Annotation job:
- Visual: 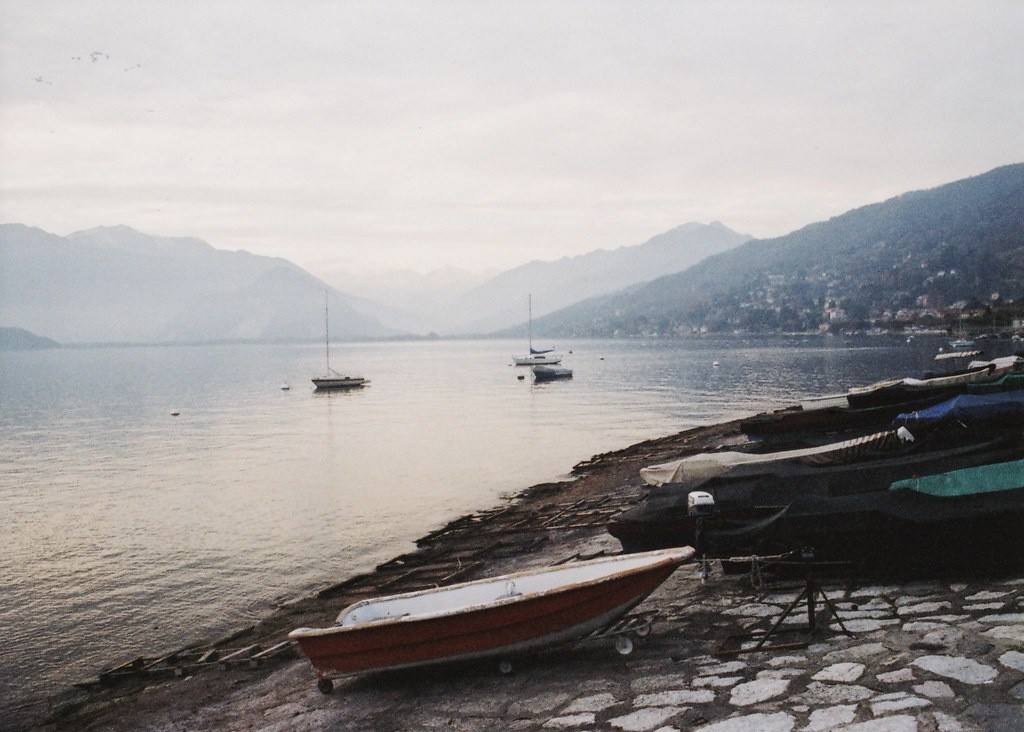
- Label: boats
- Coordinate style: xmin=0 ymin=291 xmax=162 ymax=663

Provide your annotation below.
xmin=605 ymin=309 xmax=1024 ymax=560
xmin=286 ymin=545 xmax=696 ymax=679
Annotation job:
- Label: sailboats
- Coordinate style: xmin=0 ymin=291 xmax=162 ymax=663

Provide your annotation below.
xmin=512 ymin=294 xmax=572 ymax=378
xmin=312 ymin=288 xmax=370 ymax=389
